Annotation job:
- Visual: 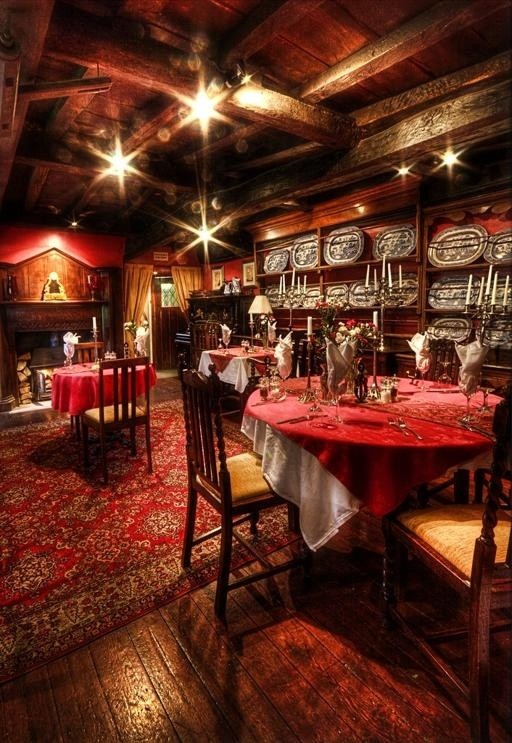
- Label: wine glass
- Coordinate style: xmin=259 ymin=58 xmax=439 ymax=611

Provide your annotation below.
xmin=457 ymin=371 xmax=496 ymax=424
xmin=63 ymin=344 xmax=74 ymax=369
xmin=437 ymin=362 xmax=452 ymax=385
xmin=307 ymin=377 xmax=349 ymax=425
xmin=217 ymin=338 xmax=224 ymax=352
xmin=417 ymin=355 xmax=432 ymax=390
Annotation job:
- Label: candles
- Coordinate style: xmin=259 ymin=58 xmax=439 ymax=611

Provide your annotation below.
xmin=279 ymin=268 xmax=307 ymax=294
xmin=365 ymin=255 xmax=402 ymax=290
xmin=92 ymin=317 xmax=97 ymax=331
xmin=466 ymin=265 xmax=510 ymax=305
xmin=250 ymin=314 xmax=253 ymax=322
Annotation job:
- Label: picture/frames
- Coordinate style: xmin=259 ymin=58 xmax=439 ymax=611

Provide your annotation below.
xmin=210 ymin=265 xmax=224 ymax=292
xmin=240 ymin=258 xmax=257 ymax=290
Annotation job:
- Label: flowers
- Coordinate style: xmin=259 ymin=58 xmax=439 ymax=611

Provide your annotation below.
xmin=254 ymin=315 xmax=276 ymax=340
xmin=309 ymin=299 xmax=383 ymax=362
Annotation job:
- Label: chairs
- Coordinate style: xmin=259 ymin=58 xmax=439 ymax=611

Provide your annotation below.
xmin=81 ymin=357 xmax=152 ymax=484
xmin=190 ymin=322 xmax=308 ymax=420
xmin=416 ymin=339 xmax=471 ymax=387
xmin=72 ymin=342 xmax=104 ymax=363
xmin=382 ymin=397 xmax=511 ymax=743
xmin=178 ymin=352 xmax=313 ymax=618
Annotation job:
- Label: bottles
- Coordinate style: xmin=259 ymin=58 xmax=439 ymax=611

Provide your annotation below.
xmin=259 ymin=358 xmax=286 ymax=403
xmin=241 ymin=340 xmax=249 ymax=352
xmin=353 ymin=364 xmax=369 ymax=401
xmin=105 ymin=349 xmax=116 ymax=359
xmin=381 ymin=376 xmax=400 ymax=405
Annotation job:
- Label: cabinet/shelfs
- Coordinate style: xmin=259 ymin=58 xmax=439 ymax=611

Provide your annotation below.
xmin=245 ymin=173 xmax=420 ymax=372
xmin=423 ymin=187 xmax=512 ymax=392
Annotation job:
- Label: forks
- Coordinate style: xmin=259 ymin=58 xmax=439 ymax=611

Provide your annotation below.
xmin=387 ymin=416 xmax=423 ymax=441
